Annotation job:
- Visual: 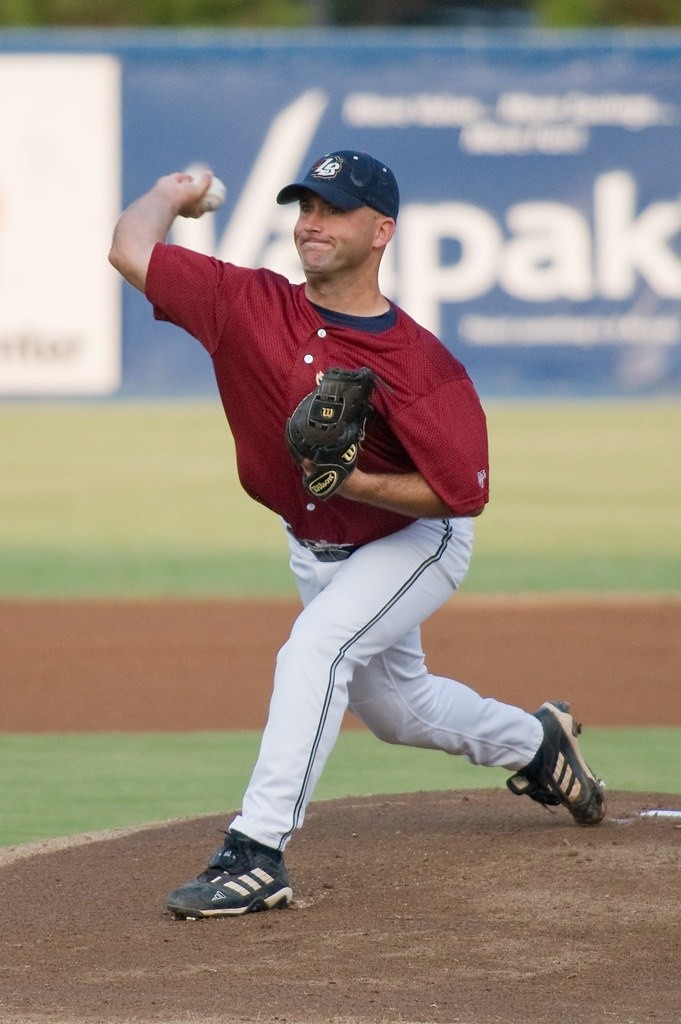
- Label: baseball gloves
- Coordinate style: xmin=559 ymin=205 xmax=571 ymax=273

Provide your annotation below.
xmin=285 ymin=366 xmax=376 ymax=500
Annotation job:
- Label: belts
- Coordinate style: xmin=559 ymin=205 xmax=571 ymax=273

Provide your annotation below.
xmin=286 ymin=526 xmax=363 ymax=562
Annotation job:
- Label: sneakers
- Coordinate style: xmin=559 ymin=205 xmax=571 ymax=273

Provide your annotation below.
xmin=506 ymin=700 xmax=607 ymax=826
xmin=167 ymin=829 xmax=293 ymax=918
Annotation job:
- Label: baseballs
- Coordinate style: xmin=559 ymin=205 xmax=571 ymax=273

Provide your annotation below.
xmin=193 ymin=176 xmax=226 ymax=211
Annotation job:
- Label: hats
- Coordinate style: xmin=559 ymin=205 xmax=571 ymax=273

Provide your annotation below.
xmin=277 ymin=149 xmax=400 ymax=220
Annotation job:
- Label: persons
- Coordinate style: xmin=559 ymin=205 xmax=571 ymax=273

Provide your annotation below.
xmin=110 ymin=149 xmax=607 ymax=918
